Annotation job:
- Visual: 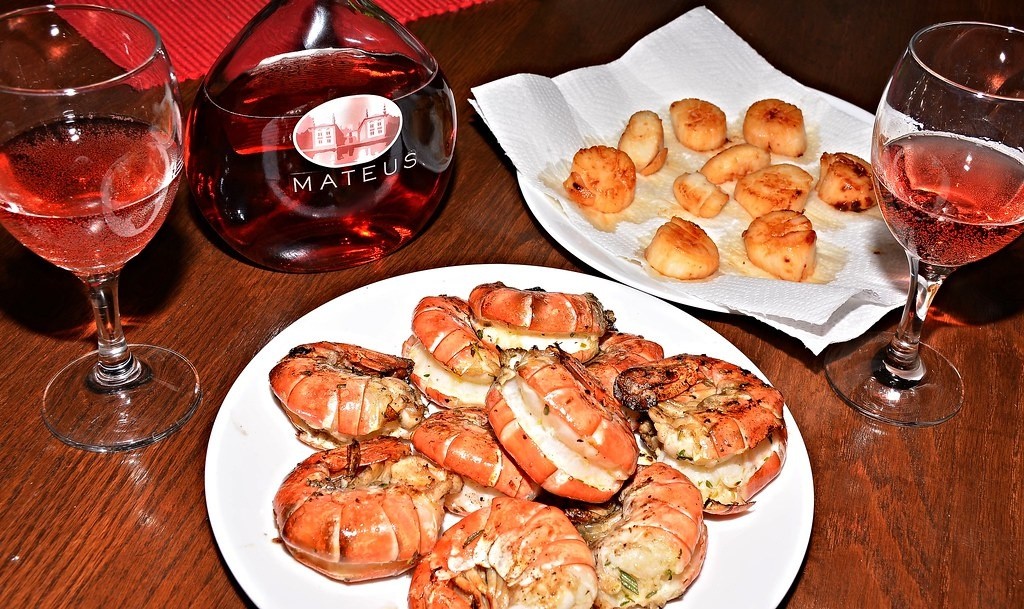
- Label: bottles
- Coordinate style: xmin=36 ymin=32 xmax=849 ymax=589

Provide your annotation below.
xmin=183 ymin=0 xmax=457 ymax=275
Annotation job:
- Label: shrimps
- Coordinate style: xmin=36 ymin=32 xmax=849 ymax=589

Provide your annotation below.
xmin=265 ymin=280 xmax=789 ymax=609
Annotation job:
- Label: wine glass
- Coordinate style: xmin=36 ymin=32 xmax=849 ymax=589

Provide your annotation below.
xmin=0 ymin=4 xmax=203 ymax=450
xmin=825 ymin=21 xmax=1024 ymax=428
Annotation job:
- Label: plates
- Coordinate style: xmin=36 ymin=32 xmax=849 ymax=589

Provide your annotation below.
xmin=204 ymin=263 xmax=815 ymax=609
xmin=517 ymin=87 xmax=877 ymax=316
